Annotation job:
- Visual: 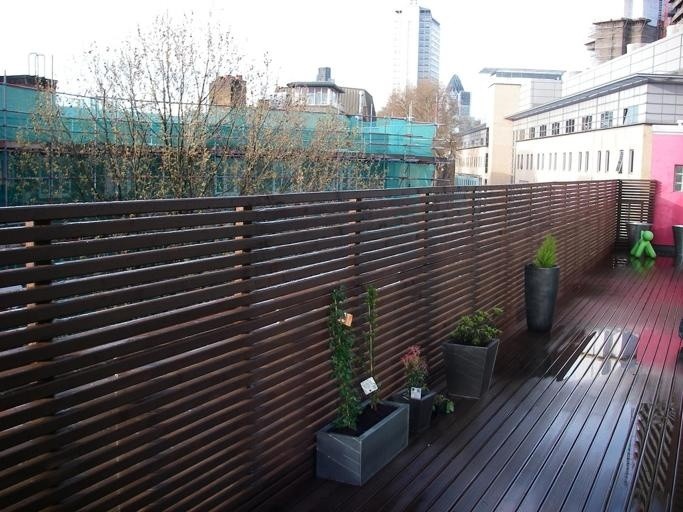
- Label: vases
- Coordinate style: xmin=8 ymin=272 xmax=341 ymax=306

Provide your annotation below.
xmin=672 ymin=225 xmax=683 ymax=256
xmin=627 ymin=223 xmax=653 ymax=256
xmin=400 ymin=390 xmax=436 ymax=434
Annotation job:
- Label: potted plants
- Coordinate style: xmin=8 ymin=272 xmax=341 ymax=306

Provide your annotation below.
xmin=525 ymin=235 xmax=561 ymax=332
xmin=442 ymin=305 xmax=504 ymax=400
xmin=317 ymin=280 xmax=409 ymax=487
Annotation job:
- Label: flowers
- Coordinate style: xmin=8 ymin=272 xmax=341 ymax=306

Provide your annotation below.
xmin=400 ymin=345 xmax=430 ymax=399
